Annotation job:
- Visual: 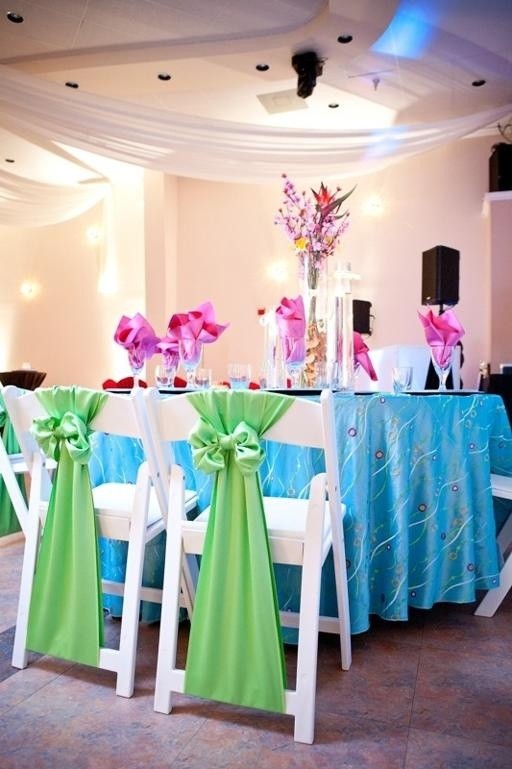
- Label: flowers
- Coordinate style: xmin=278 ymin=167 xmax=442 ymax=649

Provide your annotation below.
xmin=271 ymin=172 xmax=358 ymax=271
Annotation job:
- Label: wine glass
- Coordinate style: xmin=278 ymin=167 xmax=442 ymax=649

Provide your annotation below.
xmin=164 ymin=349 xmax=180 ymax=389
xmin=284 ymin=336 xmax=307 ymax=389
xmin=125 ymin=349 xmax=146 ymax=396
xmin=177 ymin=339 xmax=205 ymax=389
xmin=429 ymin=344 xmax=454 ymax=390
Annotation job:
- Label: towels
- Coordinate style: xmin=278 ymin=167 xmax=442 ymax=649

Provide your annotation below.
xmin=113 ymin=293 xmax=466 ymax=383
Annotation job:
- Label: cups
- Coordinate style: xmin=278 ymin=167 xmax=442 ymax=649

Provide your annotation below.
xmin=228 ymin=363 xmax=252 ymax=390
xmin=392 ymin=366 xmax=414 ymax=394
xmin=154 ymin=363 xmax=178 ymax=388
xmin=314 ymin=358 xmax=340 ymax=390
xmin=193 ymin=367 xmax=212 ymax=389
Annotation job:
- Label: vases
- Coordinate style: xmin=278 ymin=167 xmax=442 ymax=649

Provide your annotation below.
xmin=298 ymin=268 xmax=331 ymax=390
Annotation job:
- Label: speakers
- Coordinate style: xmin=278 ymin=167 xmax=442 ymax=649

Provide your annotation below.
xmin=422 ymin=245 xmax=459 ymax=305
xmin=352 ymin=299 xmax=372 ymax=335
xmin=489 ymin=145 xmax=512 ymax=191
